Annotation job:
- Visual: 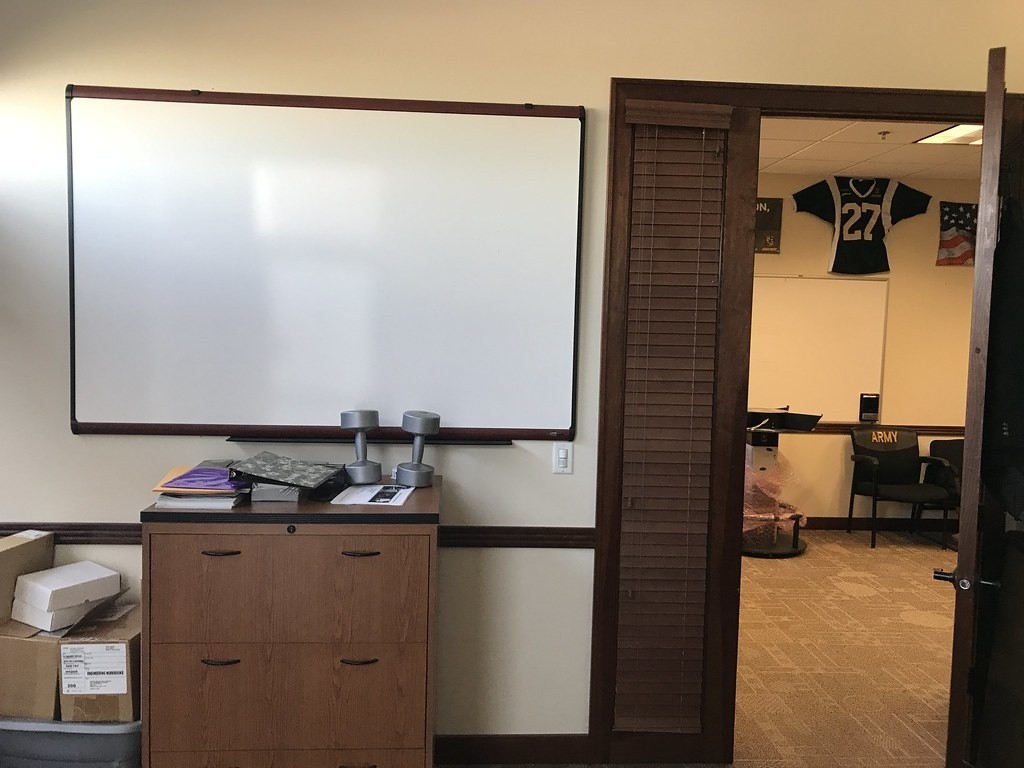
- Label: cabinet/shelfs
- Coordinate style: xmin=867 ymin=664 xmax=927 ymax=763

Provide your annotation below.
xmin=139 ymin=474 xmax=443 ymax=768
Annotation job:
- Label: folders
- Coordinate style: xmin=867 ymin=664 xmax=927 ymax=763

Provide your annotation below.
xmin=228 ymin=451 xmax=338 ymax=489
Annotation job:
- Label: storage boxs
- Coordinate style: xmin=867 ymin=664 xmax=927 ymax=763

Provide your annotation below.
xmin=746 ymin=428 xmax=779 ymax=514
xmin=59 ymin=603 xmax=142 ymax=722
xmin=0 ymin=720 xmax=142 ymax=768
xmin=0 ymin=617 xmax=73 ymax=721
xmin=0 ymin=530 xmax=57 ymax=627
xmin=10 ymin=596 xmax=113 ymax=631
xmin=14 ymin=560 xmax=120 ymax=611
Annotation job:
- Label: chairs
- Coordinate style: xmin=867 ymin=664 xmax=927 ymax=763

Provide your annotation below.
xmin=846 ymin=425 xmax=951 ymax=552
xmin=914 ymin=439 xmax=964 ymax=533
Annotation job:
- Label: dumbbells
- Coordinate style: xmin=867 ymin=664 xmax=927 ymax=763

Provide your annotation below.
xmin=396 ymin=410 xmax=440 ymax=487
xmin=341 ymin=411 xmax=383 ymax=486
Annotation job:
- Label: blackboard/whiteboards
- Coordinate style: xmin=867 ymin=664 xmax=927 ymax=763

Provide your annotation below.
xmin=65 ymin=82 xmax=585 ymax=444
xmin=747 ymin=272 xmax=891 ymax=426
